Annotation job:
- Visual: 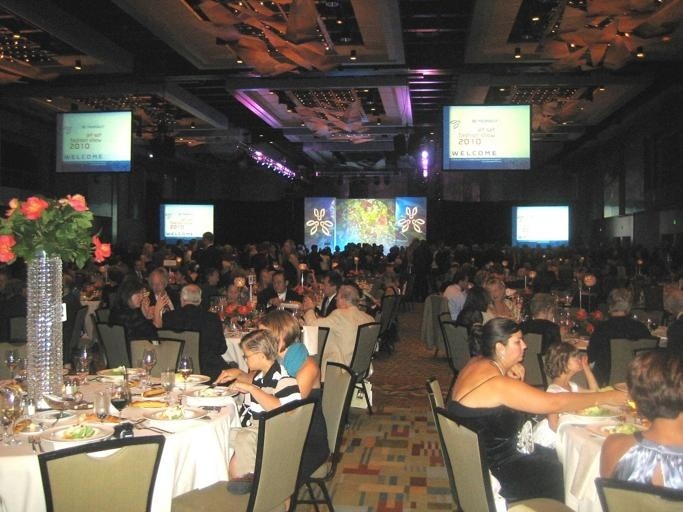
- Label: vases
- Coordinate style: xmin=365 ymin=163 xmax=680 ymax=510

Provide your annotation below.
xmin=26 ymin=260 xmax=63 ymax=403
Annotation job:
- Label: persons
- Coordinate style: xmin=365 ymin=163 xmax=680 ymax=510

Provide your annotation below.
xmin=585 ymin=286 xmax=660 ymax=386
xmin=0 ymin=257 xmax=29 ymax=343
xmin=443 ymin=273 xmax=469 ymax=322
xmin=662 ymin=289 xmax=682 ymax=356
xmin=455 ymin=287 xmax=488 ymax=358
xmin=514 ymin=293 xmax=562 ymax=359
xmin=109 ymin=275 xmax=169 ymax=343
xmin=219 ymin=239 xmax=407 ymax=322
xmin=141 ymin=254 xmax=154 ymax=272
xmin=62 ymin=242 xmax=110 ymax=375
xmin=128 ymin=257 xmax=148 ymax=281
xmin=483 ymin=277 xmax=521 ymax=324
xmin=302 ymin=286 xmax=374 ymax=384
xmin=199 ymin=269 xmax=225 ymax=312
xmin=200 ymin=232 xmax=220 ymax=269
xmin=529 ymin=341 xmax=598 ymax=449
xmin=149 ymin=267 xmax=180 ymax=315
xmin=211 ymin=311 xmax=329 ymax=512
xmin=110 ymin=238 xmax=201 ymax=262
xmin=407 ymin=237 xmax=432 ymax=302
xmin=445 ymin=316 xmax=629 ymax=505
xmin=160 ymin=284 xmax=239 ymax=385
xmin=426 ymin=235 xmax=683 ymax=291
xmin=599 ymin=349 xmax=682 ymax=492
xmin=229 ymin=329 xmax=303 ymax=482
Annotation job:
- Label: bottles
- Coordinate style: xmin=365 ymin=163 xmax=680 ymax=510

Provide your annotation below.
xmin=72 ymin=381 xmax=78 ymax=397
xmin=27 ymin=399 xmax=36 ymax=418
xmin=20 ymin=400 xmax=27 ymax=417
xmin=65 ymin=381 xmax=72 ymax=395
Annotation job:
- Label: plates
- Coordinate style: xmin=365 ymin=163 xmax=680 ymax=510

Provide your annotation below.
xmin=143 ymin=405 xmax=212 ymax=424
xmin=185 ymin=385 xmax=239 ymax=400
xmin=97 ymin=367 xmax=146 ymax=383
xmin=39 ymin=423 xmax=117 ymax=443
xmin=601 ymin=424 xmax=649 ymax=435
xmin=176 ymin=372 xmax=212 ymax=385
xmin=32 ymin=409 xmax=75 ymax=423
xmin=614 ymin=381 xmax=628 ymax=391
xmin=563 ymin=404 xmax=624 ymax=420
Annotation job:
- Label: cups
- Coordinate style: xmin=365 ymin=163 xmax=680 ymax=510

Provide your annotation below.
xmin=110 ymin=385 xmax=127 ymax=423
xmin=95 ymin=391 xmax=107 ymax=424
xmin=70 ymin=352 xmax=94 ymax=385
xmin=2 ymin=388 xmax=23 ymax=446
xmin=237 ymin=314 xmax=246 ymax=330
xmin=178 ymin=356 xmax=193 ymax=389
xmin=159 ymin=368 xmax=175 ymax=404
xmin=293 ymin=308 xmax=302 ymax=323
xmin=141 ymin=349 xmax=158 ymax=382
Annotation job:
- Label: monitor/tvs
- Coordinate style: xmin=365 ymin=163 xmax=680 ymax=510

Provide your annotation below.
xmin=441 ymin=104 xmax=532 ymax=171
xmin=56 ymin=110 xmax=133 ymax=175
xmin=159 ymin=202 xmax=215 ymax=241
xmin=510 ymin=203 xmax=573 ymax=249
xmin=303 ymin=196 xmax=428 ymax=256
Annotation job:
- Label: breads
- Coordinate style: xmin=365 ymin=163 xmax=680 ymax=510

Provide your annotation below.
xmin=128 ymin=379 xmax=139 ymax=387
xmin=85 ymin=413 xmax=120 ymax=423
xmin=128 ymin=400 xmax=169 ymax=409
xmin=143 ymin=386 xmax=166 ymax=399
xmin=13 ymin=418 xmax=32 ymax=433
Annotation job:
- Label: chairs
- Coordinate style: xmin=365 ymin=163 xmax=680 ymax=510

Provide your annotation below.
xmin=8 ymin=315 xmax=26 ymax=341
xmin=291 ymin=360 xmax=357 ymax=512
xmin=94 ymin=322 xmax=130 ymax=370
xmin=299 ymin=326 xmax=332 ymax=397
xmin=425 ymin=378 xmax=534 ymax=504
xmin=348 ymin=322 xmax=382 ymax=415
xmin=630 ymin=306 xmax=665 ymax=329
xmin=522 ymin=332 xmax=549 ymax=390
xmin=398 ymin=275 xmax=414 ymax=313
xmin=129 ymin=338 xmax=186 ymax=374
xmin=154 ymin=328 xmax=202 ymax=373
xmin=64 ymin=304 xmax=88 ymax=371
xmin=608 ymin=336 xmax=661 ymax=390
xmin=170 ymin=396 xmax=317 ymax=512
xmin=0 ymin=342 xmax=26 ymax=379
xmin=441 ymin=321 xmax=473 ymax=373
xmin=438 ymin=313 xmax=454 ymax=359
xmin=376 ymin=294 xmax=398 ymax=354
xmin=432 ymin=406 xmax=575 ymax=512
xmin=594 ymin=477 xmax=683 ymax=511
xmin=37 ymin=434 xmax=166 ymax=512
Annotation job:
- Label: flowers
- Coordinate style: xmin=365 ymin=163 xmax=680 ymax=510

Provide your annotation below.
xmin=569 ymin=308 xmax=605 ymax=336
xmin=222 ymin=302 xmax=254 ymax=329
xmin=0 ymin=196 xmax=111 ymax=259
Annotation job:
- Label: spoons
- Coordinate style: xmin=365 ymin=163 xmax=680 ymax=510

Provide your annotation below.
xmin=133 ymin=424 xmax=164 ymax=437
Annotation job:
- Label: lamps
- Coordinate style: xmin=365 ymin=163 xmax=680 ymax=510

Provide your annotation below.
xmin=600 ymin=85 xmax=605 ymax=91
xmin=14 ymin=28 xmax=20 ymax=40
xmin=636 ymin=47 xmax=643 ymax=58
xmin=190 ymin=121 xmax=196 ymax=129
xmin=351 ymin=49 xmax=357 ymax=61
xmin=515 ymin=47 xmax=522 ymax=58
xmin=337 ymin=16 xmax=342 ymax=25
xmin=74 ymin=59 xmax=83 ymax=72
xmin=533 ymin=12 xmax=540 ymax=22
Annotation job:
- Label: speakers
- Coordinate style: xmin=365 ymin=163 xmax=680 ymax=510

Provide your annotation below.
xmin=170 ymin=143 xmax=207 ymax=172
xmin=571 ymin=145 xmax=605 ymax=180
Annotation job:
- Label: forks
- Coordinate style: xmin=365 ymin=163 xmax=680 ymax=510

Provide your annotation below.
xmin=139 ymin=422 xmax=175 ymax=436
xmin=33 ymin=435 xmax=46 ymax=454
xmin=26 ymin=434 xmax=39 ymax=455
